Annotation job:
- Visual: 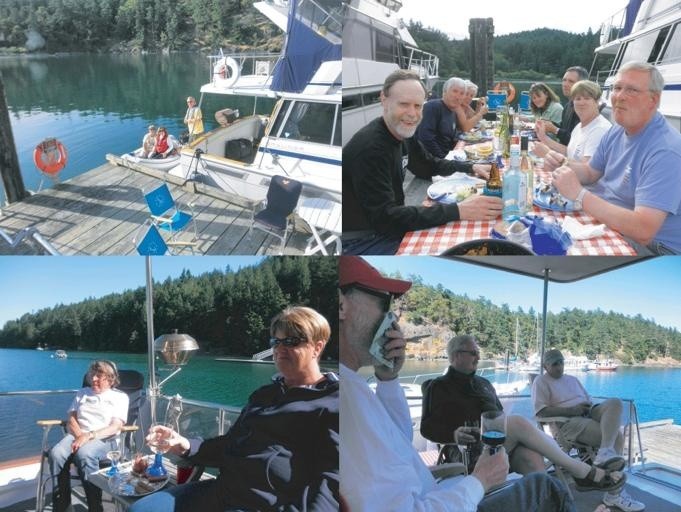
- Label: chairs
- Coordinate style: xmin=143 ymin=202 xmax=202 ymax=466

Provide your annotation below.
xmin=418 ymin=380 xmax=472 ymax=474
xmin=36 ymin=370 xmax=144 ymax=511
xmin=144 ymin=182 xmax=198 ymax=244
xmin=518 ymin=90 xmax=533 ymax=115
xmin=532 ymin=415 xmax=626 ymax=501
xmin=250 ymin=175 xmax=303 ymax=255
xmin=429 ymin=463 xmax=468 ymax=484
xmin=132 ymin=218 xmax=174 ymax=256
xmin=486 ymin=89 xmax=508 ymax=110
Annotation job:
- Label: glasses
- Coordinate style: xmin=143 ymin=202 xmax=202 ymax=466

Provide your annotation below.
xmin=342 ymin=285 xmax=396 ymax=314
xmin=452 ymin=349 xmax=481 ymax=357
xmin=269 ymin=335 xmax=308 ymax=349
xmin=612 ymin=85 xmax=656 ymax=98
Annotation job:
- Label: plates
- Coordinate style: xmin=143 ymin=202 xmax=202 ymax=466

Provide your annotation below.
xmin=107 ymin=470 xmax=170 ymax=498
xmin=454 ymin=150 xmax=498 ymax=164
xmin=427 ymin=176 xmax=486 ymax=206
xmin=458 ymin=131 xmax=493 ymax=142
xmin=528 ymin=150 xmax=543 ymax=160
xmin=533 ymin=185 xmax=581 ymax=212
xmin=439 ymin=238 xmax=537 ymax=256
xmin=521 ymin=131 xmax=540 ymax=141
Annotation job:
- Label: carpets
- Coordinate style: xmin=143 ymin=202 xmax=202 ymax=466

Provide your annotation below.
xmin=0 ymin=484 xmax=119 ymax=512
xmin=563 ymin=482 xmax=681 ymax=512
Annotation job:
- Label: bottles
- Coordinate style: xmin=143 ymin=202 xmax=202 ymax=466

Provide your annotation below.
xmin=508 ymin=107 xmax=515 ymax=116
xmin=519 ymin=134 xmax=533 ymax=211
xmin=486 ymin=161 xmax=502 ymax=220
xmin=501 ymin=134 xmax=527 ymax=223
xmin=499 ymin=112 xmax=510 ymax=159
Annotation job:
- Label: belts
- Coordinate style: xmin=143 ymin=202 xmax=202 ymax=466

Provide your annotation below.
xmin=343 ymin=228 xmax=381 ymax=240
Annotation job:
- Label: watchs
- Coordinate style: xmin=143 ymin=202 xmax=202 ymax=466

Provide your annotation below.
xmin=572 ymin=187 xmax=587 ymax=213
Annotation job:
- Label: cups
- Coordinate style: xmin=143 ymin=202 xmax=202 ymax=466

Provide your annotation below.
xmin=583 ymin=393 xmax=593 ymax=418
xmin=130 ymin=452 xmax=148 ymax=477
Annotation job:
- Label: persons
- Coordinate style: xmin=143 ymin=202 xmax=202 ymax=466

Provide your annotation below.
xmin=148 ymin=128 xmax=171 ymax=160
xmin=48 ymin=360 xmax=130 ymax=511
xmin=339 ymin=256 xmax=579 ymax=511
xmin=141 ymin=125 xmax=156 ymax=158
xmin=130 ymin=307 xmax=338 ymax=512
xmin=421 ymin=336 xmax=627 ymax=492
xmin=185 ymin=97 xmax=203 ymax=142
xmin=544 ymin=61 xmax=680 ymax=257
xmin=531 ymin=350 xmax=645 ymax=511
xmin=416 ymin=66 xmax=614 ymax=164
xmin=341 ymin=69 xmax=503 ymax=256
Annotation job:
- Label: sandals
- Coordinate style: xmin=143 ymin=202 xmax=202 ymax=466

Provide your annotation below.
xmin=574 ymin=464 xmax=628 ymax=492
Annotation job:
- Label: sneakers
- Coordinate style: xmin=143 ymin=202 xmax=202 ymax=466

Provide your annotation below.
xmin=601 ymin=488 xmax=646 ymax=511
xmin=593 ymin=447 xmax=627 ymax=474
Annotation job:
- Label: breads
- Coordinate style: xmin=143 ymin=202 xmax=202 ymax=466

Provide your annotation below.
xmin=478 ymin=144 xmax=493 ymax=156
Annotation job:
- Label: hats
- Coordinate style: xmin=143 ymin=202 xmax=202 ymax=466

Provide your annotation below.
xmin=340 ymin=255 xmax=412 ymax=297
xmin=542 ymin=348 xmax=564 ymax=367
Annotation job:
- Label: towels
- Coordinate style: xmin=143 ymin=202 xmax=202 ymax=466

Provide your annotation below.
xmin=368 ymin=312 xmax=399 ymax=372
xmin=530 ymin=215 xmax=573 ymax=256
xmin=562 ymin=213 xmax=608 ymax=245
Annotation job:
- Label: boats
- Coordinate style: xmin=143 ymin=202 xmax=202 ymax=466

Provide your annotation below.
xmin=127 ymin=140 xmax=181 ymax=169
xmin=54 ymin=347 xmax=69 ymax=361
xmin=182 ymin=0 xmax=341 ymax=234
xmin=35 ymin=341 xmax=49 ymax=351
xmin=361 ymin=365 xmax=532 ymax=448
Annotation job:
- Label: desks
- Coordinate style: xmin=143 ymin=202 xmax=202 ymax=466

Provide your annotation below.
xmin=298 ymin=198 xmax=342 ymax=257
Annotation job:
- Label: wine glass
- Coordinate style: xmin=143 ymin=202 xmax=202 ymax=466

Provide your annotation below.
xmin=106 ymin=438 xmax=122 ymax=475
xmin=464 ymin=418 xmax=479 ymax=452
xmin=143 ymin=421 xmax=172 ymax=482
xmin=496 ymin=106 xmax=505 ymax=119
xmin=479 ymin=411 xmax=507 ymax=455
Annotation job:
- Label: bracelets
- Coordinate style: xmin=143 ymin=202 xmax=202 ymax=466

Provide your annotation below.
xmin=89 ymin=431 xmax=94 ymax=438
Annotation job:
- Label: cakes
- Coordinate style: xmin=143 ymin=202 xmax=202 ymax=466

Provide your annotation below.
xmin=456 ymin=185 xmax=477 ymax=202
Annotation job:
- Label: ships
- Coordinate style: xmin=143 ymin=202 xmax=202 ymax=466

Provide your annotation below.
xmin=341 ymin=0 xmax=441 ymax=150
xmin=589 ymin=1 xmax=681 ymax=119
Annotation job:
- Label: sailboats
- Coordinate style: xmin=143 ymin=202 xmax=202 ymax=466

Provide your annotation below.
xmin=495 ymin=310 xmax=620 ymax=373
